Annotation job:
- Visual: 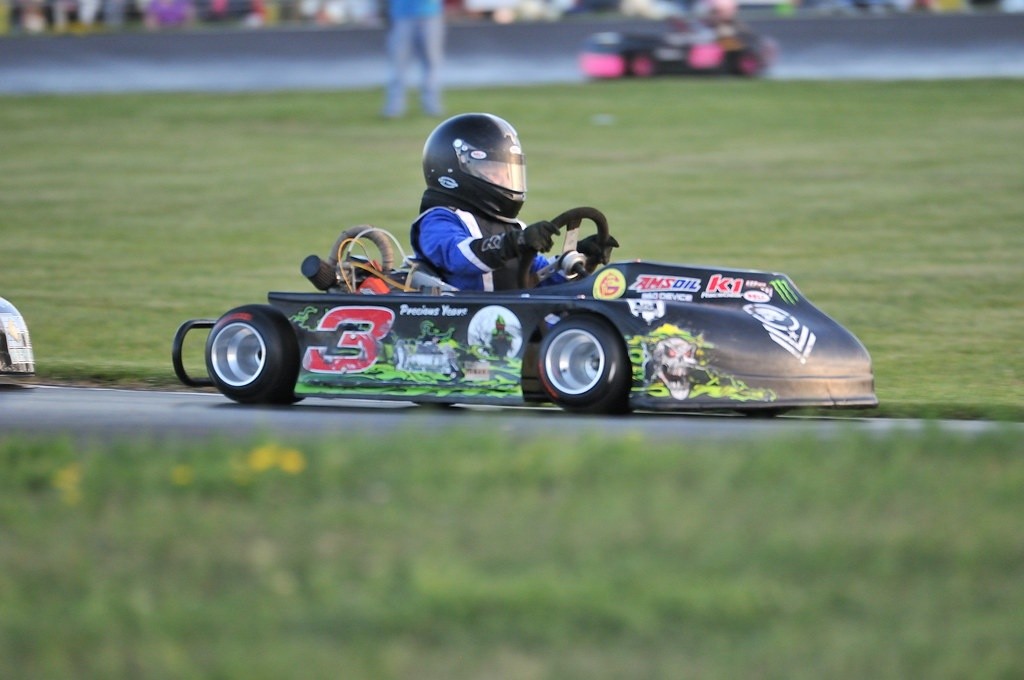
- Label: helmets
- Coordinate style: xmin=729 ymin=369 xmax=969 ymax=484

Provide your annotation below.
xmin=423 ymin=113 xmax=527 ymax=220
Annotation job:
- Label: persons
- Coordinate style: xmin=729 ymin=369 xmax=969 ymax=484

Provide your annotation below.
xmin=381 ymin=0 xmax=447 ymax=119
xmin=410 ymin=113 xmax=620 ymax=290
xmin=667 ymin=0 xmax=738 ymax=42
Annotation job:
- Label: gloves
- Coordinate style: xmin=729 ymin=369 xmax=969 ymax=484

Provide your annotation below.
xmin=576 ymin=233 xmax=619 ymax=266
xmin=507 ymin=220 xmax=560 ymax=258
